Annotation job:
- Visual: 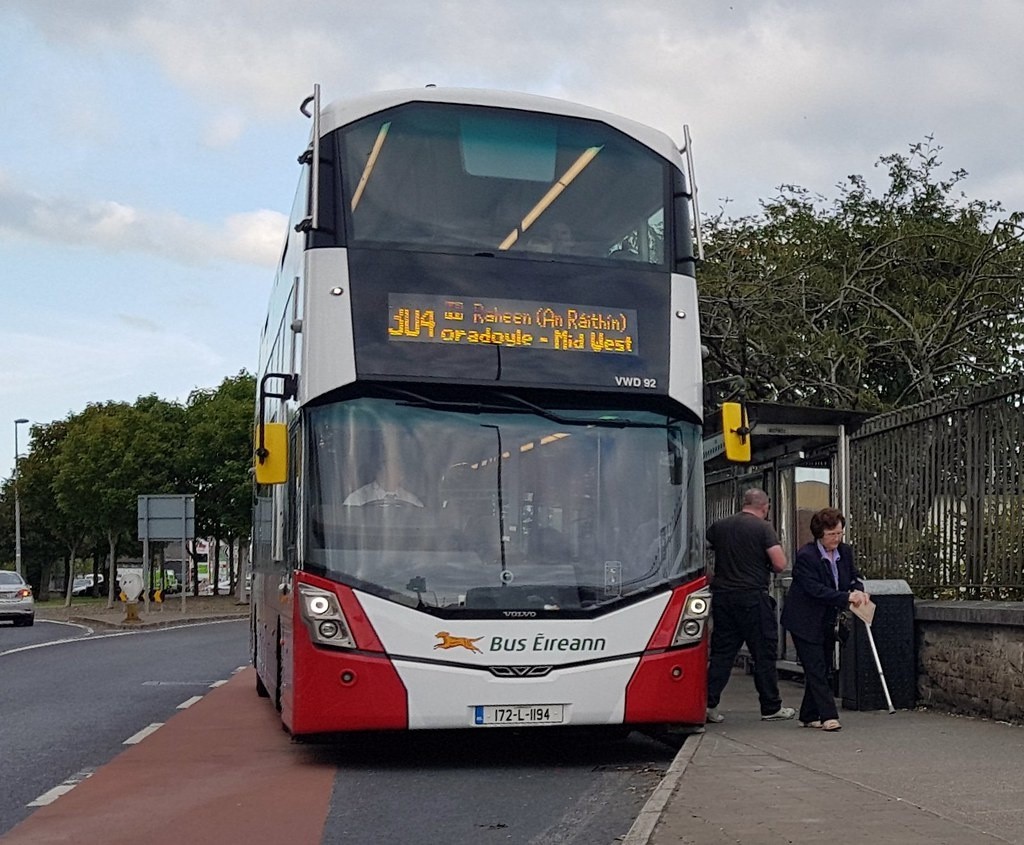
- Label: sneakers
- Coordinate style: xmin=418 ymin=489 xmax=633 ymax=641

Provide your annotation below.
xmin=706 ymin=704 xmax=725 ymax=722
xmin=761 ymin=708 xmax=795 ymax=720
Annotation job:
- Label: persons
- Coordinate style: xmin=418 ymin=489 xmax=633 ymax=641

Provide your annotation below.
xmin=780 ymin=508 xmax=871 ymax=732
xmin=704 ymin=488 xmax=789 ymax=723
xmin=343 ymin=451 xmax=427 ymax=509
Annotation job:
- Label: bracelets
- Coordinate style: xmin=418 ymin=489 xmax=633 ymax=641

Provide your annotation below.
xmin=823 ymin=544 xmax=834 ymax=552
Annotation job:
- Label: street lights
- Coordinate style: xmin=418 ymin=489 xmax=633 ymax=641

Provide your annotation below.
xmin=13 ymin=418 xmax=29 ymax=577
xmin=478 ymin=423 xmax=508 ymax=587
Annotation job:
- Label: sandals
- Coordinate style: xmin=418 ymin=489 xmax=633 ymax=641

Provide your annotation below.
xmin=798 ymin=720 xmax=823 ymax=727
xmin=823 ymin=719 xmax=842 ymax=730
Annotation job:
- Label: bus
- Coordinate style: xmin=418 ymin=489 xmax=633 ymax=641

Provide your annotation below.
xmin=244 ymin=80 xmax=764 ymax=738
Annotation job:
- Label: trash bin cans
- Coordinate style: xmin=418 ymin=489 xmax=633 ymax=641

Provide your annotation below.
xmin=840 ymin=579 xmax=916 ymax=710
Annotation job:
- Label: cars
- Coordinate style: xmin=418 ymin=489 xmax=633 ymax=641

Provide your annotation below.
xmin=0 ymin=569 xmax=36 ymax=627
xmin=70 ymin=566 xmax=145 ymax=598
